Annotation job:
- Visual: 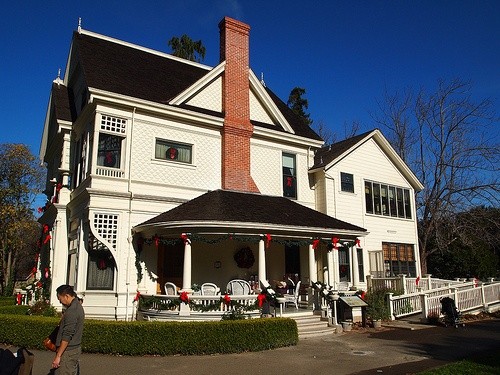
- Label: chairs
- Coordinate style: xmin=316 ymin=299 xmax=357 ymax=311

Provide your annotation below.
xmin=283 ymin=281 xmax=301 ymax=312
xmin=226 ymin=279 xmax=251 ymax=295
xmin=164 ymin=282 xmax=176 ymax=296
xmin=201 ymin=283 xmax=217 ymax=296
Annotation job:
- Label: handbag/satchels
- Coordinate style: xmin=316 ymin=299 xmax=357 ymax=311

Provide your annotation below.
xmin=43 ymin=325 xmax=60 ymax=352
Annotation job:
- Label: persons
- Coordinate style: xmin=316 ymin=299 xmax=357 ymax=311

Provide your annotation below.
xmin=16 ymin=292 xmax=22 ymax=305
xmin=51 ymin=284 xmax=85 ymax=375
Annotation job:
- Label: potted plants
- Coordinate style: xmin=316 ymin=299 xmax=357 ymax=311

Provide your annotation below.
xmin=192 ymin=283 xmax=202 ymax=296
xmin=368 ymin=290 xmax=385 ymax=328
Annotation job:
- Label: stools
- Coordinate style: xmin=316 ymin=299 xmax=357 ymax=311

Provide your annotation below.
xmin=274 ymin=298 xmax=286 ymax=318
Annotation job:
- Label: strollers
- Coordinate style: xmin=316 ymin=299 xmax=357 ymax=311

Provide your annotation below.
xmin=438 ymin=297 xmax=465 ymax=328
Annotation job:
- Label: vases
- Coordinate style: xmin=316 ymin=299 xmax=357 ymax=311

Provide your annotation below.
xmin=341 ymin=322 xmax=353 ymax=331
